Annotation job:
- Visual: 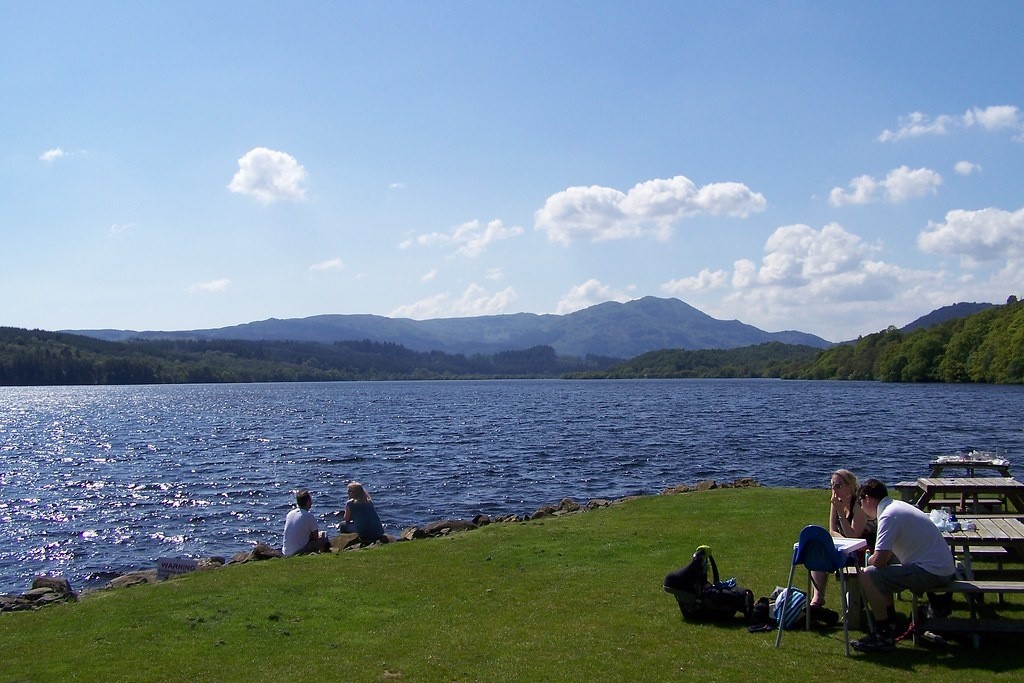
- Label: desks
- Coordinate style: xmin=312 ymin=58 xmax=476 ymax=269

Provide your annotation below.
xmin=941 ymin=516 xmax=1024 ymax=647
xmin=915 ymin=477 xmax=1024 ymax=514
xmin=928 ymin=458 xmax=1012 ymax=478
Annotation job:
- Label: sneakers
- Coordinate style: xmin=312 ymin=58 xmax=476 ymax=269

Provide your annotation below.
xmin=849 ymin=627 xmax=898 ymax=654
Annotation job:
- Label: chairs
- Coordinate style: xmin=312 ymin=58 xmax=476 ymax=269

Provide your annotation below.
xmin=775 ymin=525 xmax=874 ymax=657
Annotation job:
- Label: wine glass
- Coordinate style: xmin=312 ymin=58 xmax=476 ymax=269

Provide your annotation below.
xmin=955 ymin=451 xmax=961 ymax=462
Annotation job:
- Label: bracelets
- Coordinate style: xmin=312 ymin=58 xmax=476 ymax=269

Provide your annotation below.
xmin=839 ymin=514 xmax=845 ymax=519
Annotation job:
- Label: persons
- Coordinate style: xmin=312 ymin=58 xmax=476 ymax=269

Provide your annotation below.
xmin=850 ymin=479 xmax=956 ymax=650
xmin=803 ymin=469 xmax=883 ymax=612
xmin=340 ymin=481 xmax=385 ymax=542
xmin=282 ymin=491 xmax=328 ymax=557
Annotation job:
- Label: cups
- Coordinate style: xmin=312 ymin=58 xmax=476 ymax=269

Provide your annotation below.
xmin=942 ymin=506 xmax=951 ymax=517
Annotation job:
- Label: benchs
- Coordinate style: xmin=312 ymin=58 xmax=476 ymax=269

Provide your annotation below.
xmin=835 ymin=546 xmax=1024 ymax=646
xmin=927 ymin=499 xmax=1002 ymax=514
xmin=894 ymin=481 xmax=917 ymax=502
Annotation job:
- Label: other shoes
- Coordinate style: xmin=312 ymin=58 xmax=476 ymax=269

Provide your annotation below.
xmin=802 ymin=602 xmax=829 ymax=613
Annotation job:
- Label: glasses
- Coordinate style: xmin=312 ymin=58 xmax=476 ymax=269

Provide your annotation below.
xmin=857 ymin=496 xmax=866 ymax=505
xmin=829 ymin=481 xmax=845 ymax=489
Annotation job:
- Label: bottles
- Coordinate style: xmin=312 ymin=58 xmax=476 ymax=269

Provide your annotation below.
xmin=865 ymin=549 xmax=871 ymax=567
xmin=951 ymin=522 xmax=976 ymax=531
